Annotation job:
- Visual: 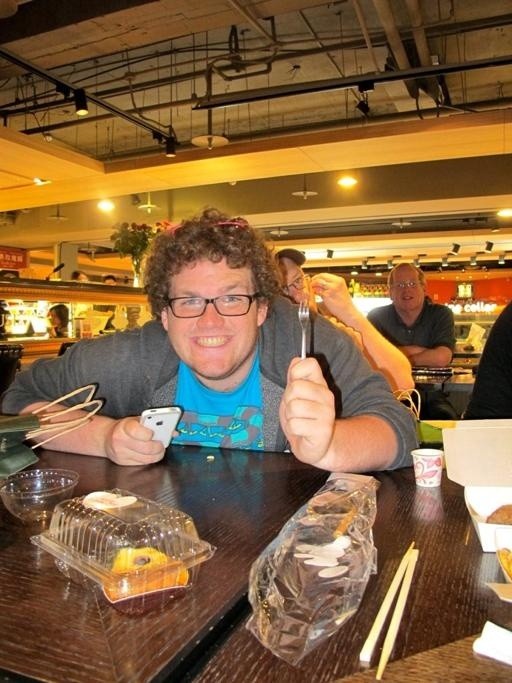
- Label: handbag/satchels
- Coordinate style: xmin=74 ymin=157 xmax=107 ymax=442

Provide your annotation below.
xmin=392 ymin=387 xmax=456 ymax=442
xmin=1 ymin=385 xmax=104 ymax=482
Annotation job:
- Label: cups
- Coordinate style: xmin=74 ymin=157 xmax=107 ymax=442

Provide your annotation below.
xmin=411 ymin=447 xmax=445 ymax=488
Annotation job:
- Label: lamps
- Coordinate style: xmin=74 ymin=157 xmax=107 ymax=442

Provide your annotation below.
xmin=138 ymin=193 xmax=159 ymax=214
xmin=73 ymin=84 xmax=89 ymax=116
xmin=165 ymin=135 xmax=178 ymax=158
xmin=485 ymin=241 xmax=493 ymax=253
xmin=269 ymin=227 xmax=290 ymax=238
xmin=360 ymin=256 xmax=506 ymax=269
xmin=391 ymin=217 xmax=411 ymax=230
xmin=190 ymin=134 xmax=231 ymax=151
xmin=292 ymin=175 xmax=318 ymax=201
xmin=451 ymin=244 xmax=460 ymax=255
xmin=326 ymin=249 xmax=334 ymax=260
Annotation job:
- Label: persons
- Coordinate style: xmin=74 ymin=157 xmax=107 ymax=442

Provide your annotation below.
xmin=276 ymin=249 xmax=416 ymax=401
xmin=45 ymin=305 xmax=71 ymax=341
xmin=71 ymin=269 xmax=94 ymax=328
xmin=1 ymin=211 xmax=418 ymax=474
xmin=364 ymin=263 xmax=462 ymax=420
xmin=98 ymin=273 xmax=117 ymax=330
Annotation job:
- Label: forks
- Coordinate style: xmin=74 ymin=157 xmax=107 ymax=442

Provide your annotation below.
xmin=296 ymin=295 xmax=311 ymax=361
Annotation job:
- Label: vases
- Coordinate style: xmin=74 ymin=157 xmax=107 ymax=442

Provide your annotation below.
xmin=133 ymin=272 xmax=143 ymax=286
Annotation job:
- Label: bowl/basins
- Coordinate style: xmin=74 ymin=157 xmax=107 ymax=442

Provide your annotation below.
xmin=0 ymin=467 xmax=82 ymax=523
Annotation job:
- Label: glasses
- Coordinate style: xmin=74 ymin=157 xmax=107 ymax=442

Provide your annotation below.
xmin=280 ymin=274 xmax=312 ymax=296
xmin=163 ymin=292 xmax=262 ymax=318
xmin=390 ymin=280 xmax=422 ymax=289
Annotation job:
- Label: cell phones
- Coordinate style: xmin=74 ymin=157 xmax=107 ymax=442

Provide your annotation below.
xmin=139 ymin=407 xmax=182 ymax=448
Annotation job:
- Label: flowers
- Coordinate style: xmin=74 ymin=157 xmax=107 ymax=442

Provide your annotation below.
xmin=112 ymin=220 xmax=167 ymax=269
